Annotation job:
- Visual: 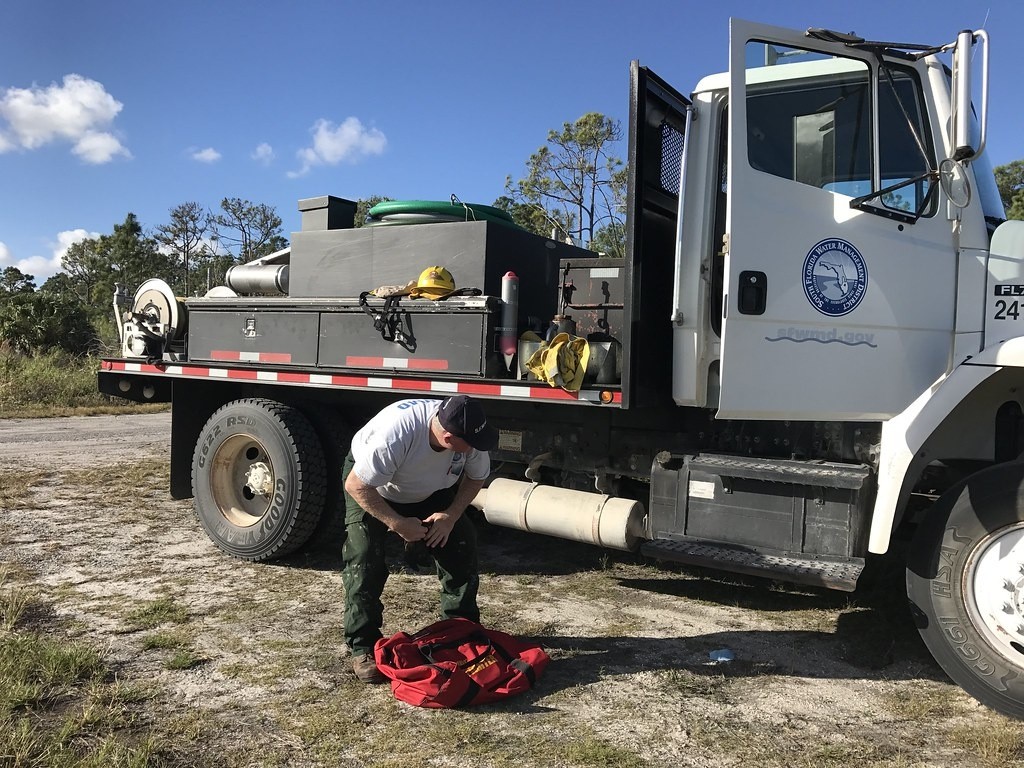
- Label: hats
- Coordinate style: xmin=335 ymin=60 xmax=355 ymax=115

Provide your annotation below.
xmin=438 ymin=395 xmax=499 ymax=451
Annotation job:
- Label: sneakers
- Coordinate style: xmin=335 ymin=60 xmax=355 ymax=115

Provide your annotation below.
xmin=353 ymin=653 xmax=383 ymax=682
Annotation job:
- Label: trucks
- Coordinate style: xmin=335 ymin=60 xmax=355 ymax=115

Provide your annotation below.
xmin=91 ymin=19 xmax=1024 ymax=726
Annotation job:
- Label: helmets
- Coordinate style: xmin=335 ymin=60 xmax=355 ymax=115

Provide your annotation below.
xmin=409 ymin=267 xmax=456 ymax=290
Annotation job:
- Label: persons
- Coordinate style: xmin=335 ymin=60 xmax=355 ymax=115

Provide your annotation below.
xmin=340 ymin=396 xmax=502 ymax=686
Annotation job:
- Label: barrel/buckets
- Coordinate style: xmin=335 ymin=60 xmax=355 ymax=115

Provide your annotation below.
xmin=517 ymin=341 xmax=543 ymax=381
xmin=583 ymin=341 xmax=617 ymax=384
